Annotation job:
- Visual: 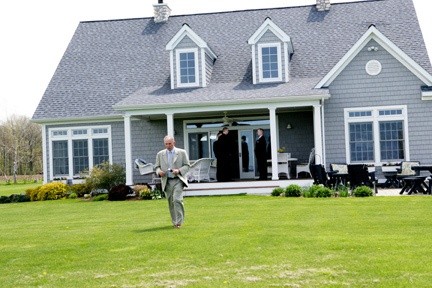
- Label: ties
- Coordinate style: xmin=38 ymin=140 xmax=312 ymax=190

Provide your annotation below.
xmin=168 ymin=150 xmax=174 ymax=178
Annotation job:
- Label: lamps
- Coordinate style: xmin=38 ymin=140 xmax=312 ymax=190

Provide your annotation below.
xmin=222 ymin=112 xmax=229 ymax=124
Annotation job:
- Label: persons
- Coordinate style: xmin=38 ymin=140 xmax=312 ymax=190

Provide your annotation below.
xmin=213 ymin=128 xmax=229 ymax=182
xmin=155 ymin=135 xmax=190 ymax=228
xmin=255 ymin=128 xmax=267 ymax=180
xmin=242 ymin=136 xmax=249 ymax=168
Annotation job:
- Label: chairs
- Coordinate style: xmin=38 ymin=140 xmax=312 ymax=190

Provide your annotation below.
xmin=277 ymin=148 xmax=420 ymax=194
xmin=135 ymin=158 xmax=214 ymax=182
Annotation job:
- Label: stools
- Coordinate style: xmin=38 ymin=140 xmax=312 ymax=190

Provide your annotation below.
xmin=399 ymin=176 xmax=431 ymax=196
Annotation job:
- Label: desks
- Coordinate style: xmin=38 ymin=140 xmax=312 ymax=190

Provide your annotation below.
xmin=266 ymin=158 xmax=298 ymax=179
xmin=411 ymin=166 xmax=432 ymax=195
xmin=332 ymin=171 xmax=378 ymax=194
